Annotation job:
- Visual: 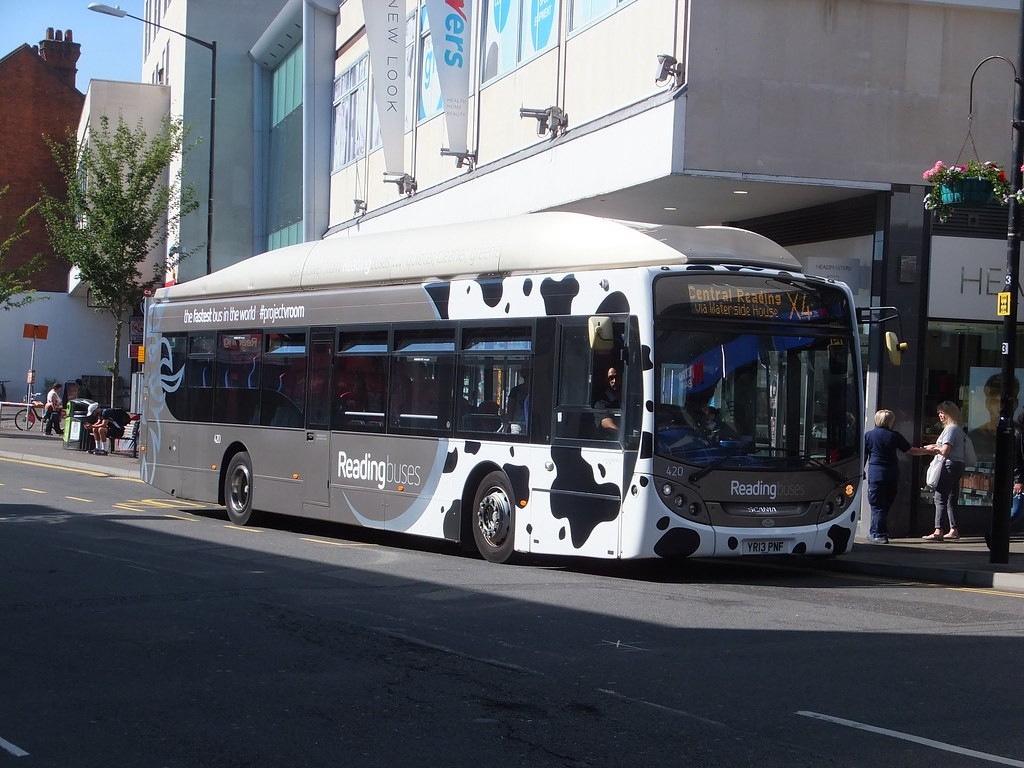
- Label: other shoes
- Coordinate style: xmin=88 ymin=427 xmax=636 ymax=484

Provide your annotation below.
xmin=866 ymin=534 xmax=888 ymax=544
xmin=943 ymin=533 xmax=959 ymax=539
xmin=922 ymin=533 xmax=944 ymax=541
xmin=86 ymin=448 xmax=109 ymax=456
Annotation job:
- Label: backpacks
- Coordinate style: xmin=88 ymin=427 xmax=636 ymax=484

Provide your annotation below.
xmin=100 ymin=408 xmax=131 ymax=427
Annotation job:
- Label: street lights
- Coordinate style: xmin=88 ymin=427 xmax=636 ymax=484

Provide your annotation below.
xmin=87 ymin=2 xmax=218 ymax=274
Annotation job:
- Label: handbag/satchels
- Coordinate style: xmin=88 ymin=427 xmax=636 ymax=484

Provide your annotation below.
xmin=926 ymin=453 xmax=946 ymax=489
xmin=963 ymin=433 xmax=977 ymax=467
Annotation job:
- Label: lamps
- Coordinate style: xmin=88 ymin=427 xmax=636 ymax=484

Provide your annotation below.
xmin=545 ymin=106 xmax=569 ymax=132
xmin=353 ymin=199 xmax=367 ymax=214
xmin=655 ymin=54 xmax=685 ymax=87
xmin=400 ymin=175 xmax=417 ymax=192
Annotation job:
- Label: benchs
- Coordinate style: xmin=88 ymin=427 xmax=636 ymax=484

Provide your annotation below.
xmin=88 ymin=421 xmax=140 ymax=457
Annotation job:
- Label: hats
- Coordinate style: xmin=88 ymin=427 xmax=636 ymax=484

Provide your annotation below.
xmin=86 ymin=402 xmax=98 ymax=416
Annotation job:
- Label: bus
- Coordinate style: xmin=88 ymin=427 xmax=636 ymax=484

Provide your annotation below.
xmin=137 ymin=213 xmax=910 ymax=575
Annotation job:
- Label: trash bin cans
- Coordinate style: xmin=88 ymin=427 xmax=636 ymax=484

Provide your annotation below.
xmin=62 ymin=398 xmax=96 ymax=451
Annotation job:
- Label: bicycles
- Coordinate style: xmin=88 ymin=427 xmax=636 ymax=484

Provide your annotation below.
xmin=15 ymin=393 xmax=69 ymax=432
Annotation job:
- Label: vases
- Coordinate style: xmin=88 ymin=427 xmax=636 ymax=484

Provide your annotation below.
xmin=939 ymin=176 xmax=994 ymax=209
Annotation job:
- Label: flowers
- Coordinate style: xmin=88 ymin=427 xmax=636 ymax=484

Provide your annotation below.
xmin=921 ymin=160 xmax=1012 ymax=223
xmin=1009 ymin=164 xmax=1024 ymax=207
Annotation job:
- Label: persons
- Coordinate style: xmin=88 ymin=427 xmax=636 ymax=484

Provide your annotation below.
xmin=74 ymin=378 xmax=87 ymax=398
xmin=84 ymin=402 xmax=124 ymax=455
xmin=920 ymin=401 xmax=966 ymax=541
xmin=842 ymin=411 xmax=856 ymax=449
xmin=985 ymin=411 xmax=1024 ymax=551
xmin=507 ymin=368 xmax=535 ymax=432
xmin=43 ymin=384 xmax=64 ymax=435
xmin=968 ymin=373 xmax=1019 ymax=461
xmin=594 ymin=366 xmax=622 ymax=432
xmin=863 ymin=409 xmax=939 ymax=544
